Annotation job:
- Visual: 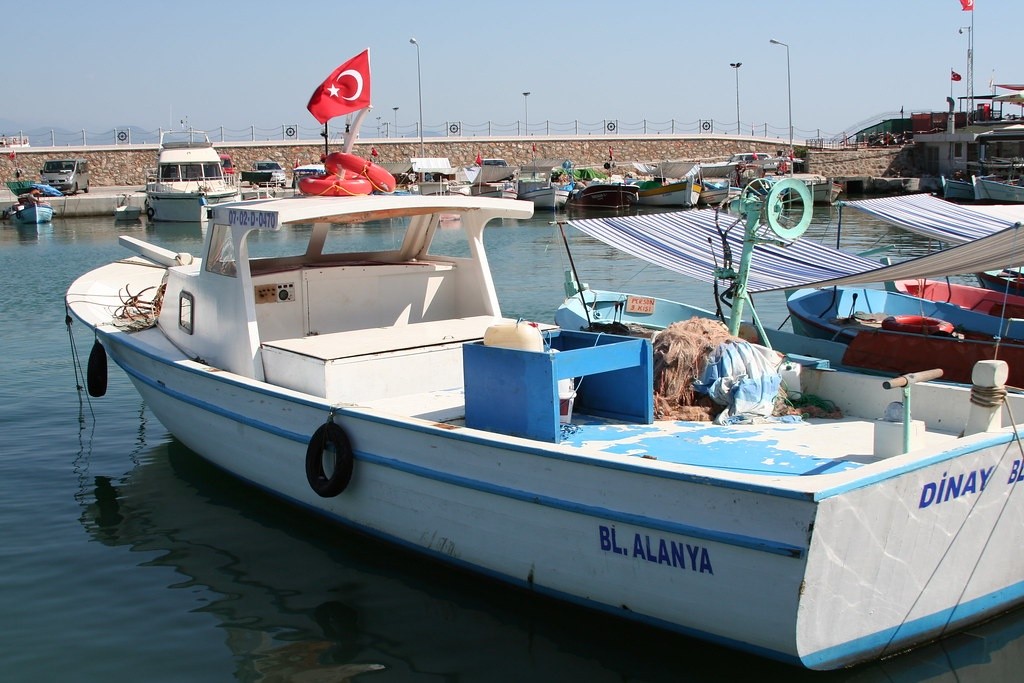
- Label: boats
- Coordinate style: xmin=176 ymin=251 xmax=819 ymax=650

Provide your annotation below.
xmin=567 ymin=180 xmax=641 ymax=210
xmin=0 ymin=135 xmax=30 ymax=149
xmin=702 ymin=178 xmax=746 ymax=208
xmin=135 ymin=118 xmax=243 ymax=222
xmin=114 ymin=193 xmax=145 ymax=222
xmin=726 ymin=184 xmax=784 ymax=223
xmin=518 ymin=142 xmax=573 ymax=213
xmin=459 ymin=157 xmax=520 ymax=200
xmin=2 ymin=189 xmax=59 ymax=226
xmin=630 ymin=175 xmax=704 ymax=208
xmin=63 ymin=72 xmax=1024 ymax=681
xmin=774 ymin=172 xmax=842 ymax=209
xmin=404 ymin=156 xmax=460 ymax=197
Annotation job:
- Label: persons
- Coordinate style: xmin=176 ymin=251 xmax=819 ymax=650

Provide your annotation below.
xmin=31 ymin=187 xmax=39 ymax=194
xmin=952 ymin=169 xmax=964 ymax=181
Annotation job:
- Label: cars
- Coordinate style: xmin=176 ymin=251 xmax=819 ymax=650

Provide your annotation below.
xmin=251 ymin=161 xmax=288 ymax=187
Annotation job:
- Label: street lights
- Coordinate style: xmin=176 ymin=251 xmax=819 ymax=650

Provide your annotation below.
xmin=767 ymin=37 xmax=803 ymax=182
xmin=959 ymin=25 xmax=975 ymax=126
xmin=410 ymin=38 xmax=428 ymax=183
xmin=730 ymin=62 xmax=743 ymax=135
xmin=392 ymin=106 xmax=399 ymax=137
xmin=521 ymin=91 xmax=530 ymax=136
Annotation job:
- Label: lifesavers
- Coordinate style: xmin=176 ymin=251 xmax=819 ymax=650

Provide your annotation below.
xmin=297 ymin=175 xmax=372 ymax=196
xmin=146 ymin=207 xmax=155 ymax=217
xmin=304 ymin=423 xmax=355 ymax=499
xmin=881 ymin=314 xmax=954 ymax=336
xmin=324 ymin=152 xmax=397 ymax=193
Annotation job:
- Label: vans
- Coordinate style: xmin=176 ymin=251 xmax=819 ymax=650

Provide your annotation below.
xmin=40 ymin=158 xmax=93 ymax=196
xmin=217 ymin=152 xmax=238 ymax=184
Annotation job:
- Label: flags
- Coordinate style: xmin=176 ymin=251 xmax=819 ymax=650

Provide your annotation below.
xmin=951 ymin=71 xmax=961 ymax=81
xmin=609 ymin=146 xmax=613 ymax=157
xmin=307 ymin=48 xmax=370 ymax=125
xmin=960 ymin=0 xmax=974 ymax=11
xmin=372 ymin=147 xmax=378 ymax=157
xmin=476 ymin=154 xmax=481 ymax=167
xmin=10 ymin=151 xmax=15 ymax=159
